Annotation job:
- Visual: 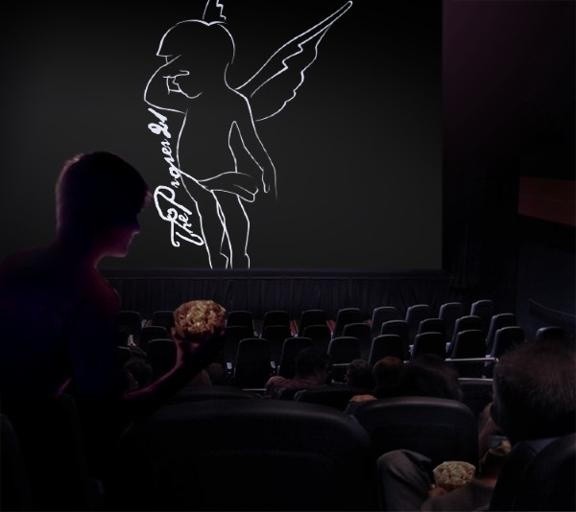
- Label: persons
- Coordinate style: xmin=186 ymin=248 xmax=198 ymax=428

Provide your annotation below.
xmin=0 ymin=152 xmax=208 ymax=511
xmin=159 ymin=327 xmax=575 ymax=511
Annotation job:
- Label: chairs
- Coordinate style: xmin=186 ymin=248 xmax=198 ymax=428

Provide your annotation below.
xmin=456 ymin=378 xmax=494 ymax=418
xmin=490 ymin=326 xmax=526 ymax=358
xmin=356 ymin=398 xmax=479 ymax=475
xmin=448 ymin=315 xmax=481 ymax=357
xmin=486 ymin=312 xmax=516 ymax=354
xmin=410 ymin=332 xmax=443 ymax=360
xmin=451 ymin=329 xmax=486 ymax=357
xmin=405 ymin=304 xmax=433 ymax=326
xmin=535 ymin=325 xmax=562 ymax=341
xmin=439 ymin=302 xmax=465 ymax=342
xmin=418 ymin=318 xmax=446 ymax=354
xmin=470 ymin=300 xmax=494 ymax=339
xmin=440 ymin=357 xmax=496 ymax=378
xmin=513 ymin=435 xmax=576 ymax=512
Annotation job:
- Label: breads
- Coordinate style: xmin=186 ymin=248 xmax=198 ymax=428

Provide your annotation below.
xmin=173 ymin=299 xmax=227 ymax=340
